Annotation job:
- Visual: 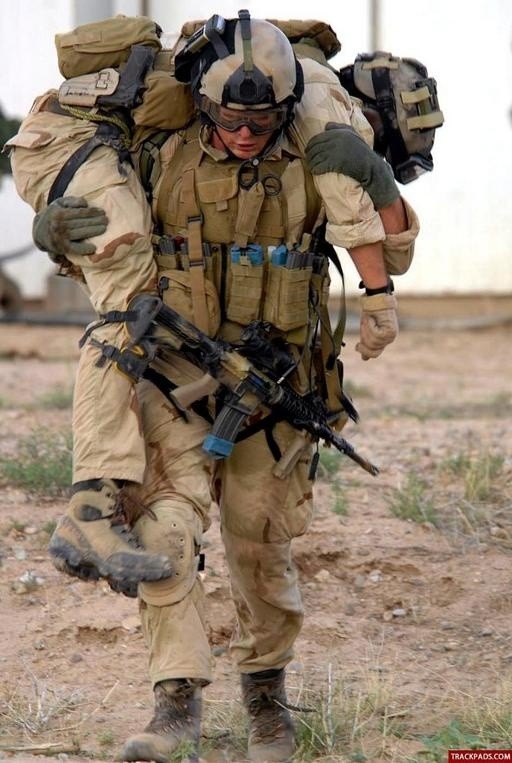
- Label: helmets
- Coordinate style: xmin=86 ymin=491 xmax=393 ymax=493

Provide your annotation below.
xmin=197 ymin=18 xmax=296 ymax=110
xmin=353 ymin=51 xmax=436 ymax=166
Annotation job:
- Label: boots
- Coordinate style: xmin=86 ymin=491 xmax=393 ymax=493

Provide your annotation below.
xmin=47 ymin=475 xmax=173 ymax=599
xmin=242 ymin=669 xmax=297 ymax=763
xmin=120 ymin=679 xmax=202 ymax=762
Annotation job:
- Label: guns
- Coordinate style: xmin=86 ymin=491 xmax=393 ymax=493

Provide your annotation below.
xmin=96 ymin=45 xmax=154 ymax=109
xmin=148 ymin=299 xmax=380 ymax=481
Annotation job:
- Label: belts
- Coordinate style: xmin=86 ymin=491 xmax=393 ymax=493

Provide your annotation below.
xmin=33 ymin=95 xmax=108 ymax=125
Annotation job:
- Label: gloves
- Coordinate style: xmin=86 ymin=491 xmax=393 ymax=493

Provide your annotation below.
xmin=32 ymin=196 xmax=109 ymax=255
xmin=356 ymin=292 xmax=399 ymax=361
xmin=305 ymin=124 xmax=400 ymax=209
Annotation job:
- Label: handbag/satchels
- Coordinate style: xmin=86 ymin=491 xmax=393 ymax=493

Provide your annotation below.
xmin=55 ymin=14 xmax=162 ymax=80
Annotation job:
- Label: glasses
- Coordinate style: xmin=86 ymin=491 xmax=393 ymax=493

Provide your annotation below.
xmin=195 ymin=89 xmax=292 ymax=136
xmin=372 ymin=51 xmax=433 ymax=186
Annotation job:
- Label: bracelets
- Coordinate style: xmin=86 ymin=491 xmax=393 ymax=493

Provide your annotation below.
xmin=359 ymin=278 xmax=394 ymax=297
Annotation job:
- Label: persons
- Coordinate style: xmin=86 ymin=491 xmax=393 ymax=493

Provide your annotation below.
xmin=0 ymin=16 xmax=448 ymax=602
xmin=31 ymin=10 xmax=421 ymax=762
